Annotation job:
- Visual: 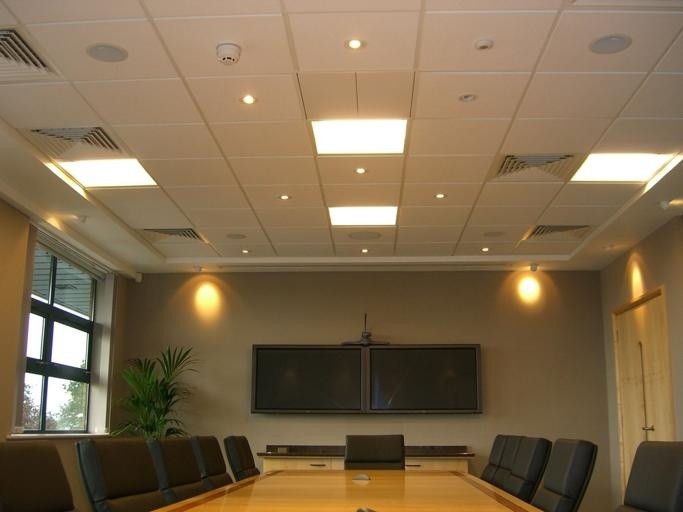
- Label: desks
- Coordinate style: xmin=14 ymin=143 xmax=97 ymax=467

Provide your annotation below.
xmin=146 ymin=469 xmax=539 ymax=512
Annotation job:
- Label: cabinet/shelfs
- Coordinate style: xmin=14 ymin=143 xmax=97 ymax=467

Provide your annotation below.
xmin=256 ymin=444 xmax=475 ymax=472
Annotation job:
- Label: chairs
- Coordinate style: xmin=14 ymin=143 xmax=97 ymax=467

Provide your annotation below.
xmin=480 ymin=433 xmax=683 ymax=512
xmin=0 ymin=435 xmax=260 ymax=512
xmin=344 ymin=433 xmax=406 ymax=469
